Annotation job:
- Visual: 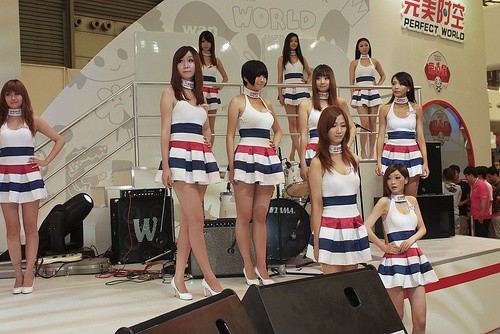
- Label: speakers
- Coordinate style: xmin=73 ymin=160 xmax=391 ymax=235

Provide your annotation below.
xmin=416 ymin=141 xmax=444 ymax=197
xmin=114 ymin=288 xmax=262 ymax=334
xmin=240 ymin=264 xmax=409 ymax=334
xmin=191 ymin=219 xmax=256 ymax=281
xmin=111 ymin=197 xmax=174 ymax=265
xmin=373 ymin=194 xmax=456 ymax=240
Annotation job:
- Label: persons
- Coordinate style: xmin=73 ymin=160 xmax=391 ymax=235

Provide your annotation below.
xmin=276 ymin=32 xmax=314 ymax=161
xmin=0 ymin=79 xmax=66 ymax=294
xmin=306 ymin=106 xmax=372 ymax=278
xmin=225 ymin=60 xmax=284 ymax=285
xmin=349 ymin=38 xmax=386 ymax=159
xmin=155 ymin=45 xmax=224 ymax=300
xmin=373 ymin=71 xmax=430 ymax=197
xmin=199 ymin=31 xmax=228 ymax=148
xmin=299 ymin=64 xmax=357 ymax=182
xmin=364 ymin=163 xmax=439 ymax=334
xmin=442 ymin=161 xmax=500 ymax=240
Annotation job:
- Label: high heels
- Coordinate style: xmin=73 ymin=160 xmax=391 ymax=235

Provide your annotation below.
xmin=21 ymin=274 xmax=35 ymax=293
xmin=242 ymin=267 xmax=259 ymax=285
xmin=202 ymin=278 xmax=221 ymax=297
xmin=254 ymin=265 xmax=276 ymax=285
xmin=12 ymin=286 xmax=23 ymax=293
xmin=171 ymin=277 xmax=192 ymax=300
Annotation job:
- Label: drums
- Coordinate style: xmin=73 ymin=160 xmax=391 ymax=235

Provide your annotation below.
xmin=219 ymin=191 xmax=237 ymax=218
xmin=264 ymin=198 xmax=311 ymax=264
xmin=283 ymin=160 xmax=311 ymax=196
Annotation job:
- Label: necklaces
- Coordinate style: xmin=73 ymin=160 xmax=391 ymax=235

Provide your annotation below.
xmin=360 ymin=54 xmax=369 ymax=59
xmin=394 ymin=96 xmax=408 ymax=104
xmin=8 ymin=108 xmax=22 ymax=116
xmin=391 ymin=193 xmax=407 ymax=202
xmin=289 ymin=50 xmax=297 ymax=56
xmin=329 ymin=144 xmax=342 ymax=154
xmin=243 ymin=85 xmax=259 ymax=98
xmin=181 ymin=79 xmax=195 ymax=89
xmin=318 ymin=92 xmax=330 ymax=99
xmin=201 ymin=50 xmax=211 ymax=56
